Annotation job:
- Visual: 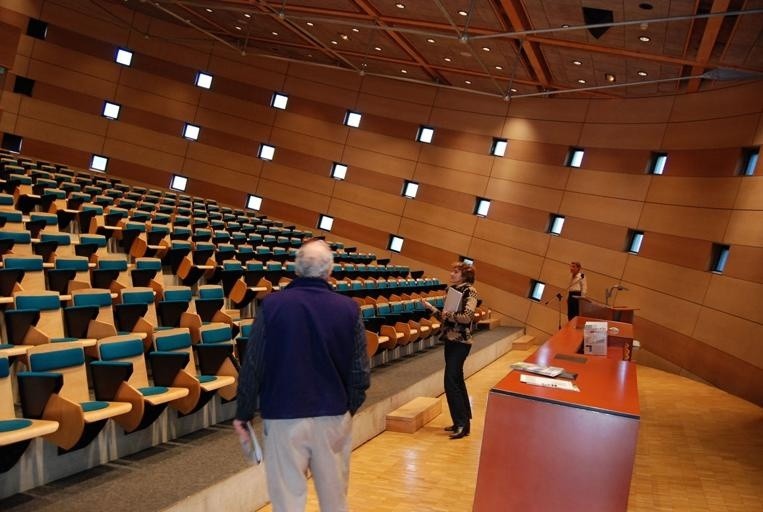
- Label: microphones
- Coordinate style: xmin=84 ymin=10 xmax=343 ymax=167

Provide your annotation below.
xmin=580 ymin=273 xmax=585 ymax=278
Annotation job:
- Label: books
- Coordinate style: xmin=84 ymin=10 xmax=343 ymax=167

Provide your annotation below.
xmin=443 ymin=286 xmax=462 ymax=312
xmin=510 ymin=361 xmax=564 ymax=378
xmin=238 ymin=422 xmax=261 ymax=465
xmin=583 ymin=321 xmax=608 ymax=355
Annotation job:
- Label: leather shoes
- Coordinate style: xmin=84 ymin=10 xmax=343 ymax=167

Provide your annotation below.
xmin=445 ymin=425 xmax=455 ymax=431
xmin=449 ymin=426 xmax=467 ymax=438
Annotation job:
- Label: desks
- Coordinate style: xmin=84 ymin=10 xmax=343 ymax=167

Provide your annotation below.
xmin=472 ymin=315 xmax=640 ymax=512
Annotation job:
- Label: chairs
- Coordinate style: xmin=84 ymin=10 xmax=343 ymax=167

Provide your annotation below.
xmin=472 ymin=298 xmax=491 ymax=321
xmin=1 ymin=194 xmax=165 ymax=341
xmin=165 ymin=191 xmax=292 ymax=328
xmin=193 ymin=321 xmax=243 ymax=405
xmin=329 ymin=240 xmax=350 ymax=295
xmin=149 ymin=327 xmax=234 ymax=418
xmin=364 ymin=239 xmax=445 ymax=357
xmin=350 ymin=241 xmax=364 ymax=305
xmin=15 ymin=342 xmax=133 ymax=455
xmin=91 ymin=332 xmax=190 ymax=433
xmin=234 ymin=318 xmax=256 ymax=369
xmin=0 ymin=146 xmax=158 ymax=193
xmin=292 ymin=224 xmax=313 ymax=273
xmin=0 ymin=356 xmax=58 ymax=472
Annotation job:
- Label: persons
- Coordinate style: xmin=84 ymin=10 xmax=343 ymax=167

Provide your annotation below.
xmin=567 ymin=262 xmax=586 ymax=320
xmin=418 ymin=260 xmax=478 ymax=438
xmin=232 ymin=240 xmax=370 ymax=512
